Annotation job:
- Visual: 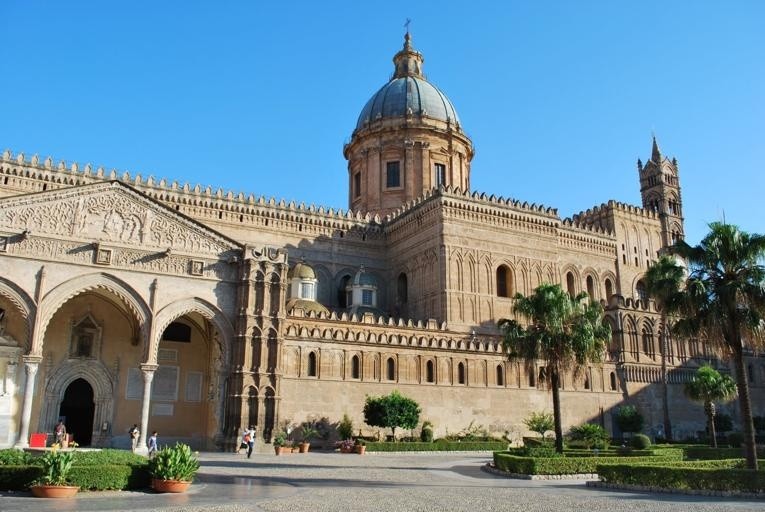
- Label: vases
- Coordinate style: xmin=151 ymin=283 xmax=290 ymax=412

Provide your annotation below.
xmin=148 ymin=477 xmax=193 ymax=493
xmin=341 ymin=445 xmax=366 ymax=454
xmin=30 ymin=484 xmax=81 ymax=499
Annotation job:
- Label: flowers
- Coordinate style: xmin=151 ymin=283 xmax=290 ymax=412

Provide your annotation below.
xmin=25 ymin=440 xmax=78 ymax=486
xmin=341 ymin=439 xmax=355 ymax=449
xmin=138 ymin=439 xmax=205 ymax=482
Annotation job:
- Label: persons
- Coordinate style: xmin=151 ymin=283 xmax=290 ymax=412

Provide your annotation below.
xmin=147 ymin=431 xmax=158 ymax=453
xmin=53 ymin=419 xmax=66 ymax=449
xmin=127 ymin=424 xmax=141 ymax=452
xmin=237 ymin=427 xmax=249 ymax=455
xmin=242 ymin=425 xmax=256 ymax=459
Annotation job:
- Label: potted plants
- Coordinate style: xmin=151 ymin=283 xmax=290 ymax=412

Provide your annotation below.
xmin=272 ymin=427 xmax=324 ymax=456
